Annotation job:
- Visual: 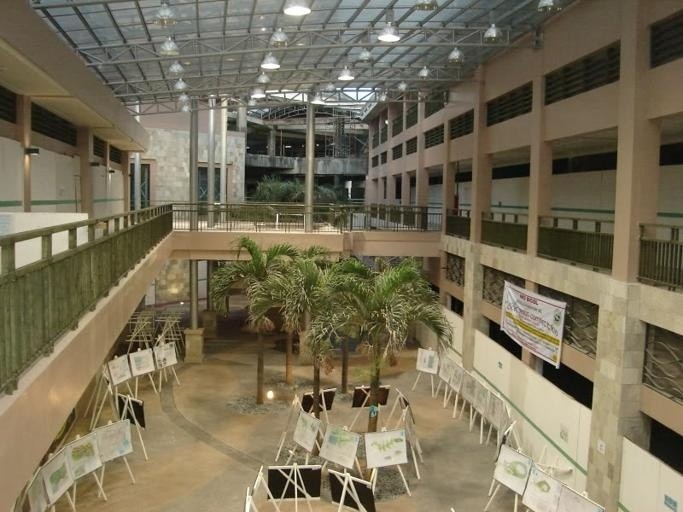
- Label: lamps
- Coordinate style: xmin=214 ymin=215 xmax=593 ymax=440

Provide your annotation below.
xmin=256 ymin=71 xmax=271 ymax=85
xmin=250 ymin=87 xmax=268 ymax=101
xmin=482 ymin=24 xmax=504 ymax=45
xmin=259 ymin=52 xmax=281 ymax=71
xmin=172 ymin=78 xmax=185 ymax=89
xmin=109 ymin=169 xmax=116 ymax=174
xmin=417 ymin=66 xmax=434 ymax=81
xmin=357 ymin=47 xmax=375 ymax=67
xmin=321 ymin=82 xmax=338 ymax=96
xmin=378 ymin=94 xmax=394 ymax=105
xmin=396 ymin=81 xmax=410 ymax=96
xmin=25 ymin=146 xmax=40 ymax=156
xmin=268 ymin=27 xmax=289 ymax=46
xmin=537 ymin=2 xmax=558 ymax=17
xmin=157 ymin=35 xmax=181 ymax=58
xmin=336 ymin=65 xmax=355 ymax=83
xmin=167 ymin=60 xmax=185 ymax=76
xmin=88 ymin=161 xmax=100 ymax=168
xmin=156 ymin=1 xmax=178 ymax=31
xmin=449 ymin=46 xmax=464 ymax=66
xmin=377 ymin=21 xmax=400 ymax=44
xmin=178 ymin=96 xmax=191 ymax=114
xmin=281 ymin=2 xmax=315 ymax=18
